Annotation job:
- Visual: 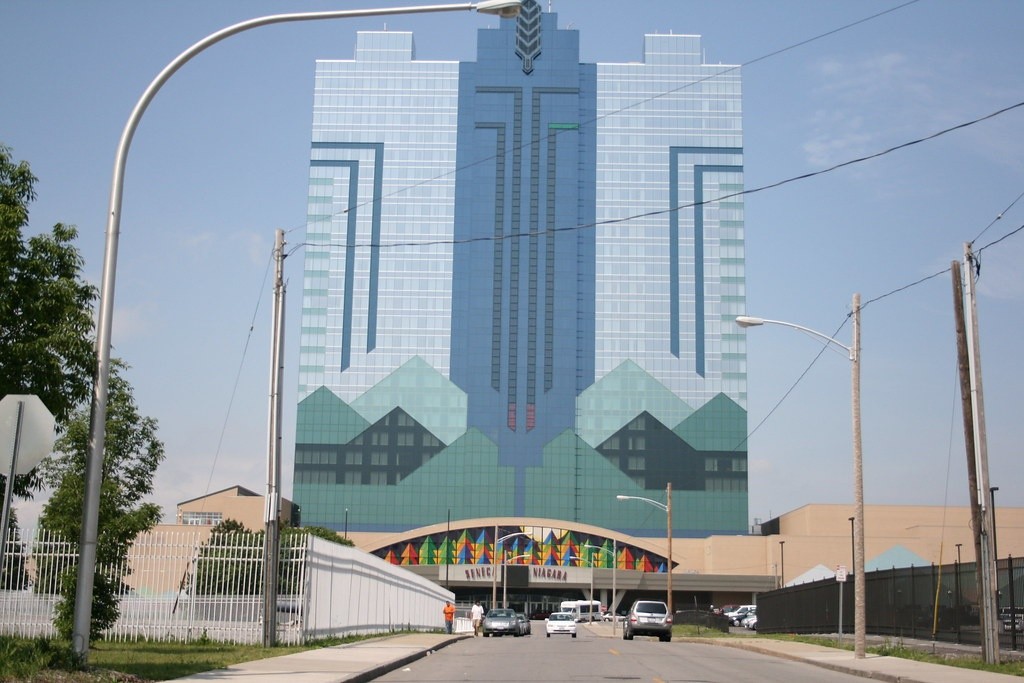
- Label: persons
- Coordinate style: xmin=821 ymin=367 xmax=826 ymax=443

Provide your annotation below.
xmin=443 ymin=601 xmax=455 ymax=634
xmin=471 ymin=599 xmax=485 ymax=636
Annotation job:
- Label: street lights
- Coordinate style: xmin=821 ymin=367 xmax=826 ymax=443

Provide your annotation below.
xmin=571 ymin=556 xmax=594 ymax=623
xmin=779 ymin=541 xmax=785 ymax=589
xmin=503 ymin=550 xmax=530 ymax=609
xmin=848 ymin=517 xmax=855 ymax=582
xmin=954 ymin=543 xmax=964 ymax=604
xmin=735 ymin=293 xmax=866 ymax=658
xmin=990 ymin=487 xmax=999 ymax=624
xmin=492 ymin=524 xmax=533 ymax=612
xmin=583 ymin=544 xmax=616 ymax=632
xmin=66 ymin=1 xmax=525 ymax=665
xmin=615 ymin=482 xmax=673 ymax=617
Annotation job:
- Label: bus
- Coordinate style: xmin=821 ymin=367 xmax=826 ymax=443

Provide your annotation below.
xmin=561 ymin=599 xmax=603 ymax=622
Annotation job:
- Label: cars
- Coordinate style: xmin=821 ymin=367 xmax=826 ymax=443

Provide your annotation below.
xmin=545 ymin=612 xmax=577 ymax=638
xmin=481 ymin=609 xmax=532 ymax=638
xmin=621 ymin=600 xmax=673 ymax=642
xmin=603 ymin=613 xmax=625 ymax=621
xmin=1001 ymin=606 xmax=1024 ymax=631
xmin=709 ymin=604 xmax=760 ymax=630
